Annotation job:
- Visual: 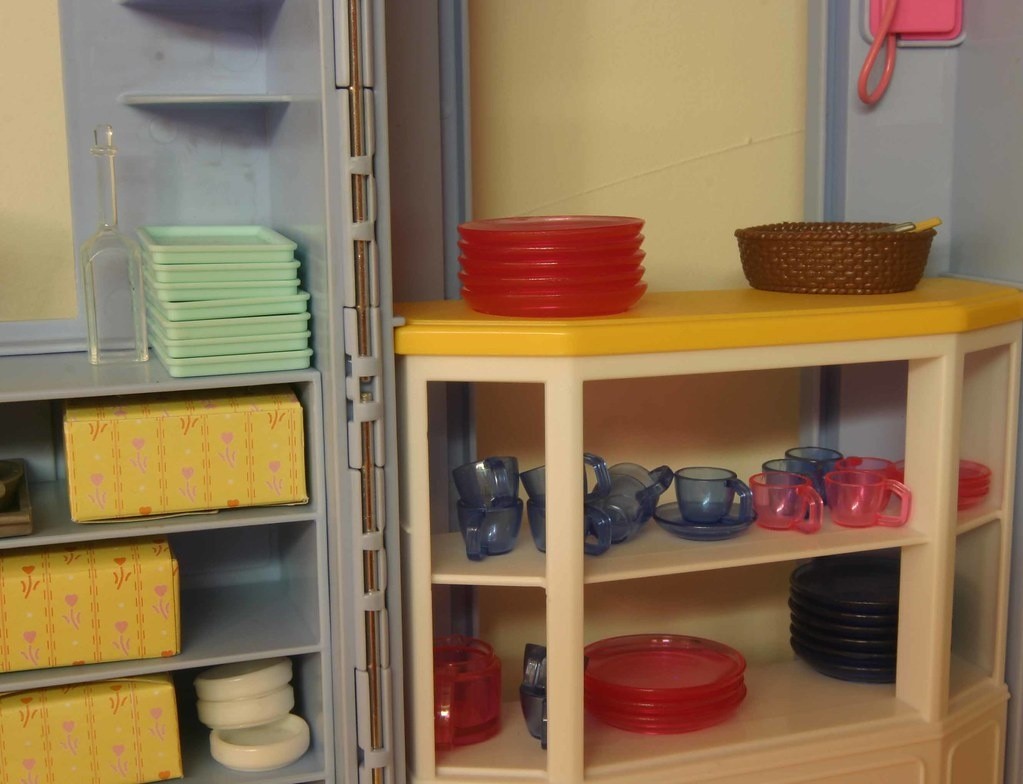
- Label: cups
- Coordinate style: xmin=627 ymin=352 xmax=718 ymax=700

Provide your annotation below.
xmin=749 ymin=472 xmax=824 ymax=535
xmin=673 ymin=467 xmax=752 ymax=525
xmin=784 ymin=446 xmax=844 ymax=504
xmin=835 ymin=456 xmax=895 ymax=511
xmin=825 ymin=470 xmax=912 ymax=528
xmin=451 ymin=453 xmax=611 ymax=562
xmin=761 ymin=458 xmax=827 ymax=519
xmin=518 ymin=643 xmax=589 ymax=750
xmin=589 ymin=462 xmax=673 ymax=544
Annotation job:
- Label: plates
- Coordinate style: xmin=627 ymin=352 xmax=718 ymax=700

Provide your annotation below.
xmin=653 ymin=502 xmax=760 ymax=541
xmin=894 ymin=458 xmax=992 ymax=511
xmin=456 ymin=215 xmax=647 ymax=318
xmin=135 ymin=227 xmax=316 ymax=378
xmin=584 ymin=633 xmax=747 ymax=734
xmin=787 ymin=555 xmax=901 ymax=684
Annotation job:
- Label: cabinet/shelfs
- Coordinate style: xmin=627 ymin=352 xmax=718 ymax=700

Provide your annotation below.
xmin=397 ymin=273 xmax=1017 ymax=784
xmin=1 ymin=347 xmax=337 ymax=783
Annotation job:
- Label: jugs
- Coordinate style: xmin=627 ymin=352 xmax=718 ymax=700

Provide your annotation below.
xmin=433 ymin=633 xmax=503 ymax=753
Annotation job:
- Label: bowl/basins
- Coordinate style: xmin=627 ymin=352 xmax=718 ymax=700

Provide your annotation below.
xmin=194 ymin=656 xmax=310 ymax=773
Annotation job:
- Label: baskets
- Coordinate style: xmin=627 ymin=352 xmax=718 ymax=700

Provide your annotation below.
xmin=734 ymin=222 xmax=937 ymax=295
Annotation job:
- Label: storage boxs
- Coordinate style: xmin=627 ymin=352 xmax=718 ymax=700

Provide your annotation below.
xmin=3 ymin=676 xmax=183 ymax=784
xmin=64 ymin=390 xmax=308 ymax=526
xmin=0 ymin=540 xmax=181 ymax=675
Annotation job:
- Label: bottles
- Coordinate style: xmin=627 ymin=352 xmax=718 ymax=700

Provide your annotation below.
xmin=79 ymin=124 xmax=149 ymax=365
